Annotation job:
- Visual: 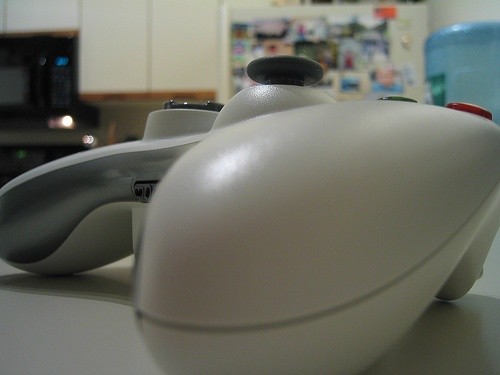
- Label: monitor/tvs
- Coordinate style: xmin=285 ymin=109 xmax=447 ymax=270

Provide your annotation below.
xmin=0 ymin=30 xmax=78 ymax=120
